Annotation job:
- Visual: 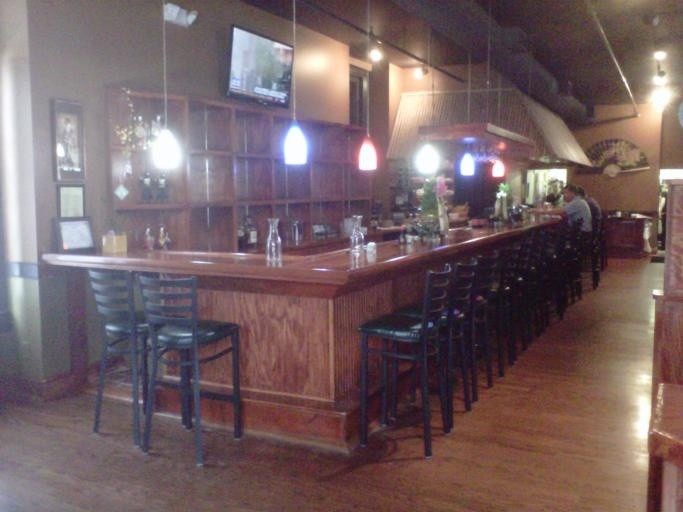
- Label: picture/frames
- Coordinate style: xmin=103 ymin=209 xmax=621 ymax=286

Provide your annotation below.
xmin=58 ymin=184 xmax=85 ymax=220
xmin=52 ymin=217 xmax=97 ymax=254
xmin=50 ymin=97 xmax=87 ymax=185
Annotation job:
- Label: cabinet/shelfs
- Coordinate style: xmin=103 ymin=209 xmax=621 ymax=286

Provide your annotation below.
xmin=103 ymin=91 xmax=380 ymax=257
xmin=603 ymin=214 xmax=652 ymax=258
xmin=652 ymin=179 xmax=683 ymax=386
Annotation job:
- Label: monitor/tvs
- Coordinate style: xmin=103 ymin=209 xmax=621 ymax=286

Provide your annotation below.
xmin=227 ymin=25 xmax=293 ymax=108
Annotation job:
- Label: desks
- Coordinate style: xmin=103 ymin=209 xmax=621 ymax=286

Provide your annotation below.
xmin=646 ymin=382 xmax=683 ymax=511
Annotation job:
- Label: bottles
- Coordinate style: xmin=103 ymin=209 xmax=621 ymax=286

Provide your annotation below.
xmin=142 ymin=170 xmax=168 ymax=203
xmin=238 ymin=215 xmax=283 ymax=260
xmin=398 ymin=225 xmax=407 ymax=244
xmin=350 ymin=215 xmax=365 ymax=254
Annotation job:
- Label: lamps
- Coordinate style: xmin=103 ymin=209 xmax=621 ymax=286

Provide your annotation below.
xmin=147 ymin=0 xmax=184 ymax=174
xmin=490 ymin=71 xmax=507 ymax=178
xmin=283 ymin=0 xmax=309 ymax=168
xmin=415 ymin=24 xmax=442 ymax=175
xmin=363 ymin=24 xmax=386 ymax=63
xmin=356 ymin=1 xmax=379 ymax=172
xmin=458 ymin=52 xmax=477 ymax=178
xmin=641 ymin=13 xmax=670 ymax=88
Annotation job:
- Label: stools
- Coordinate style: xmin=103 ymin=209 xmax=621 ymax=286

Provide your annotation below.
xmin=471 ymin=218 xmax=606 ymax=378
xmin=358 ymin=264 xmax=449 ymax=459
xmin=391 ymin=258 xmax=480 ymax=430
xmin=439 ymin=248 xmax=499 ymax=403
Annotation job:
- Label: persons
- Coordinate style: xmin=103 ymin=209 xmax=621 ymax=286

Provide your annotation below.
xmin=58 ymin=117 xmax=74 ymax=168
xmin=540 ymin=180 xmax=562 ymax=207
xmin=573 ymin=186 xmax=602 ymax=242
xmin=522 ymin=184 xmax=592 ymax=254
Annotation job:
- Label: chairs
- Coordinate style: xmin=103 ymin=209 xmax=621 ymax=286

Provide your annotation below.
xmin=134 ymin=272 xmax=241 ymax=466
xmin=88 ymin=267 xmax=188 ymax=444
